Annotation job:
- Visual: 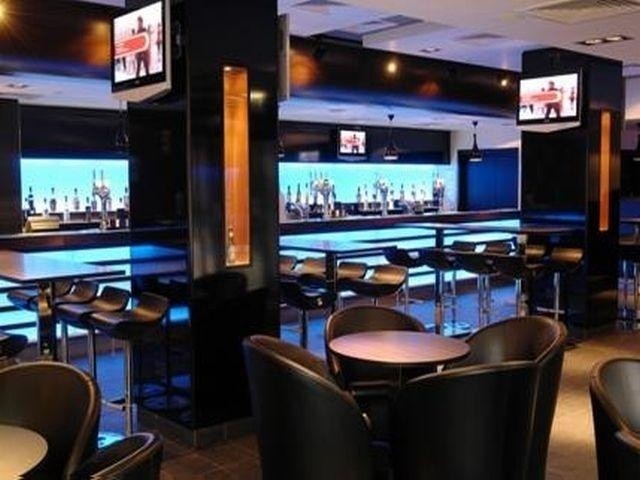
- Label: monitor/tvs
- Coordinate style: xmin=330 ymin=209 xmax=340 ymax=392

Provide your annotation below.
xmin=516 ymin=64 xmax=584 ymax=135
xmin=110 ymin=1 xmax=172 ymax=105
xmin=336 ymin=125 xmax=368 ymax=162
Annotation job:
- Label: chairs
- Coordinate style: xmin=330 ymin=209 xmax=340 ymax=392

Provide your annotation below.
xmin=589 ymin=354 xmax=640 ymax=479
xmin=0 ymin=359 xmax=162 ymax=480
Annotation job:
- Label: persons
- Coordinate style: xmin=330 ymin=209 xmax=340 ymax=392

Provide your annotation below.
xmin=543 ymin=81 xmax=563 ymax=117
xmin=133 ymin=16 xmax=150 ymax=78
xmin=351 ymin=133 xmax=360 ymax=153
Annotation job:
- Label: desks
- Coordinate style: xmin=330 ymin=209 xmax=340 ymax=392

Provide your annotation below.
xmin=0 ymin=245 xmax=131 ymax=287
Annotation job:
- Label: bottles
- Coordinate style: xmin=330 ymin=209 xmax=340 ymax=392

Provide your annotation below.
xmin=278 ymin=171 xmax=449 ymax=220
xmin=21 ymin=169 xmax=129 ymax=230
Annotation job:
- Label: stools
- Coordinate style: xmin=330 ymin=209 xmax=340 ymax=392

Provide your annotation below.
xmin=0 ymin=279 xmax=171 ymax=435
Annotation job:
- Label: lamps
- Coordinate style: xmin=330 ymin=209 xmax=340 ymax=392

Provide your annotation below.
xmin=383 ymin=114 xmax=398 ymax=160
xmin=468 ymin=120 xmax=483 ymax=161
xmin=113 ymin=97 xmax=130 ymax=157
xmin=277 ymin=131 xmax=285 ymax=157
